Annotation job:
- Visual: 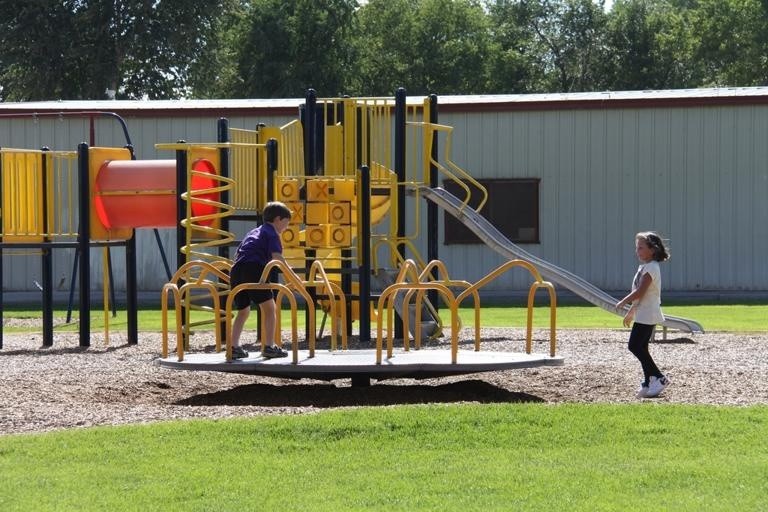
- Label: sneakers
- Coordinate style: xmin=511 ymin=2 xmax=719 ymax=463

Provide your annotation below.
xmin=230 ymin=346 xmax=248 ymax=358
xmin=645 ymin=374 xmax=669 ymax=396
xmin=636 ymin=382 xmax=650 ymax=398
xmin=263 ymin=344 xmax=288 ymax=357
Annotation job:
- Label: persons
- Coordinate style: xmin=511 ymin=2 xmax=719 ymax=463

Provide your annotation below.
xmin=613 ymin=232 xmax=671 ymax=398
xmin=226 ymin=202 xmax=304 ymax=359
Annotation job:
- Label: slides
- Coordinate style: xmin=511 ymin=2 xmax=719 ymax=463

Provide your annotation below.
xmin=281 ymin=158 xmax=399 ymax=321
xmin=421 ymin=185 xmax=705 ymax=333
